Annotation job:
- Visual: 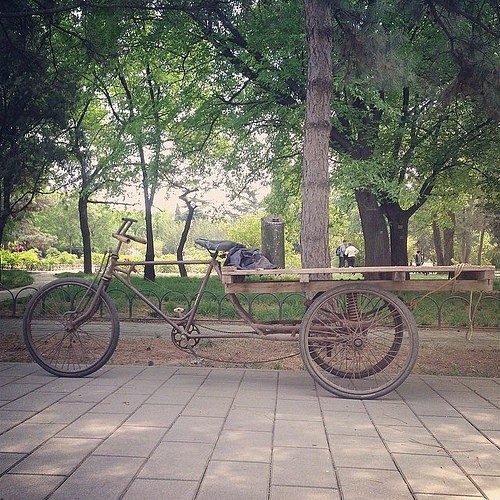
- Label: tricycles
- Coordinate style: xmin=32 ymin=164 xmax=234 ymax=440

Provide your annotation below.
xmin=21 ymin=217 xmax=495 ymax=400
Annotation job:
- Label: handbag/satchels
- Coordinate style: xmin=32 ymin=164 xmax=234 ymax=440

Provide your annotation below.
xmin=223 ymin=243 xmax=278 ymax=270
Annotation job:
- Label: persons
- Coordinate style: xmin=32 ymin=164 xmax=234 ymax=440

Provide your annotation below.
xmin=0 ymin=243 xmax=4 ymax=249
xmin=344 ymin=243 xmax=359 ymax=267
xmin=415 ymin=251 xmax=424 ymax=266
xmin=15 ymin=244 xmax=24 ymax=252
xmin=338 ymin=240 xmax=348 ymax=268
